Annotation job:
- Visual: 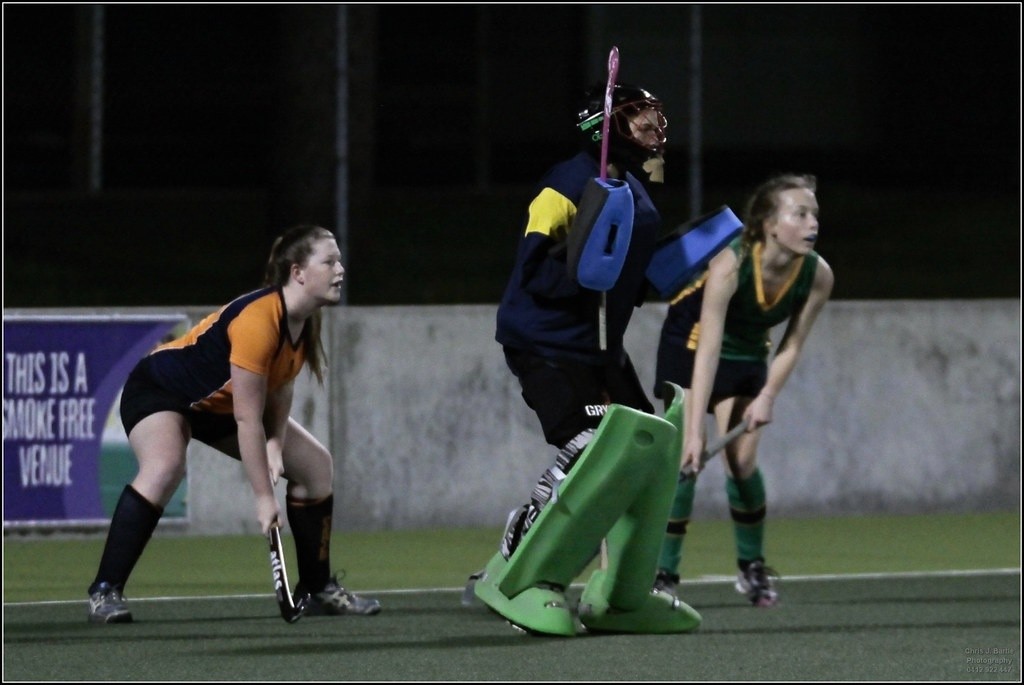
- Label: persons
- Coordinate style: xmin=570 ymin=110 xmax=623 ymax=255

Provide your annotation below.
xmin=87 ymin=223 xmax=381 ymax=624
xmin=474 ymin=80 xmax=746 ymax=636
xmin=653 ymin=173 xmax=833 ymax=610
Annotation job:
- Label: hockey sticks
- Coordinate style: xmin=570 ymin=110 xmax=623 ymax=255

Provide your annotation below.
xmin=463 ymin=414 xmax=746 ymax=606
xmin=269 ymin=521 xmax=311 ymax=625
xmin=598 ymin=45 xmax=619 ymax=350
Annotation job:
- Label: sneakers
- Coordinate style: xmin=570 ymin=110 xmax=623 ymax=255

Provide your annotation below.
xmin=294 ymin=573 xmax=380 ymax=617
xmin=734 ymin=556 xmax=782 ymax=612
xmin=87 ymin=582 xmax=133 ymax=622
xmin=654 ymin=567 xmax=681 ymax=596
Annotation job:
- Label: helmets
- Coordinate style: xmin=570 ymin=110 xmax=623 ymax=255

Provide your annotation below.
xmin=578 ymin=84 xmax=669 ymax=170
